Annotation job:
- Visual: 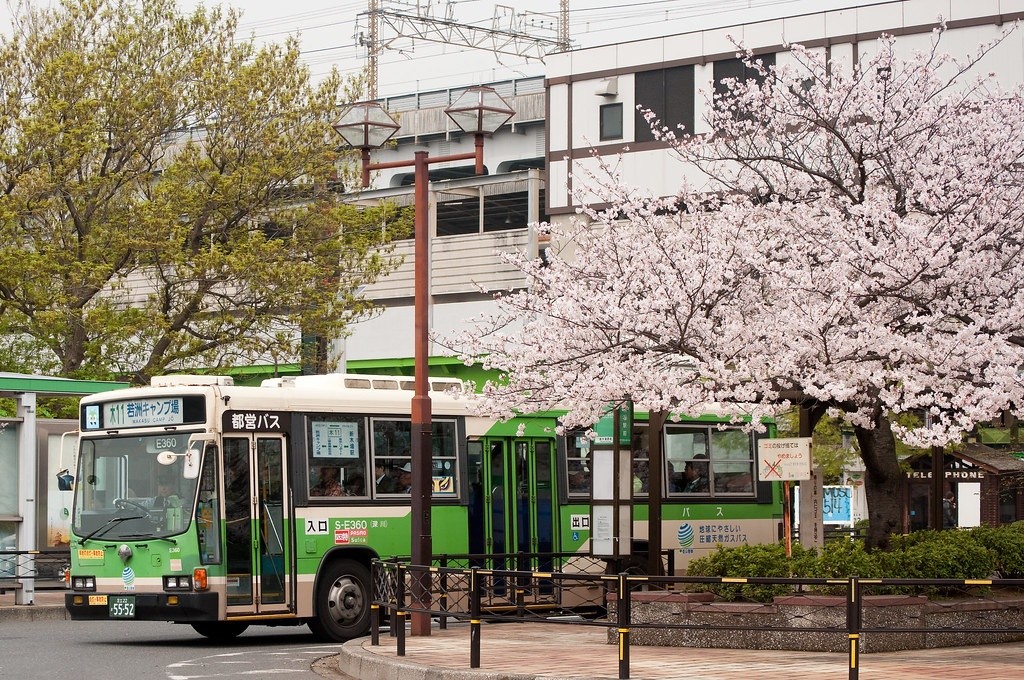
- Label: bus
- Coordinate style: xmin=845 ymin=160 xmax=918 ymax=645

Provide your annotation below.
xmin=55 ymin=373 xmax=785 ymax=644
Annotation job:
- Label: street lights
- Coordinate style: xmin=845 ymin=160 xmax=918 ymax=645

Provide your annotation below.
xmin=331 ymin=86 xmax=517 ymax=636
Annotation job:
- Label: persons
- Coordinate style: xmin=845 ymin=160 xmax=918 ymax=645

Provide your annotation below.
xmin=943 ymin=491 xmax=957 ymax=528
xmin=310 ymin=466 xmax=345 ymax=497
xmin=225 ymin=458 xmax=249 ymax=501
xmin=375 ymin=459 xmax=395 ymax=495
xmin=112 ymin=474 xmax=181 ymax=510
xmin=398 ymin=464 xmax=412 ymax=494
xmin=633 ymin=452 xmax=709 ymax=492
xmin=574 ymin=452 xmax=592 ymax=495
xmin=346 ymin=472 xmax=365 ymax=496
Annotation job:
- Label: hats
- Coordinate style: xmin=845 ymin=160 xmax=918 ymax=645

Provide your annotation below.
xmin=156 ymin=472 xmax=176 ymax=484
xmin=400 ymin=463 xmax=411 ymax=472
xmin=363 ymin=454 xmax=389 ymax=467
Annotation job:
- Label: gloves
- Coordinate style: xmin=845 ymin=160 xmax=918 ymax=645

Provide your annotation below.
xmin=113 ymin=497 xmax=126 ymax=510
